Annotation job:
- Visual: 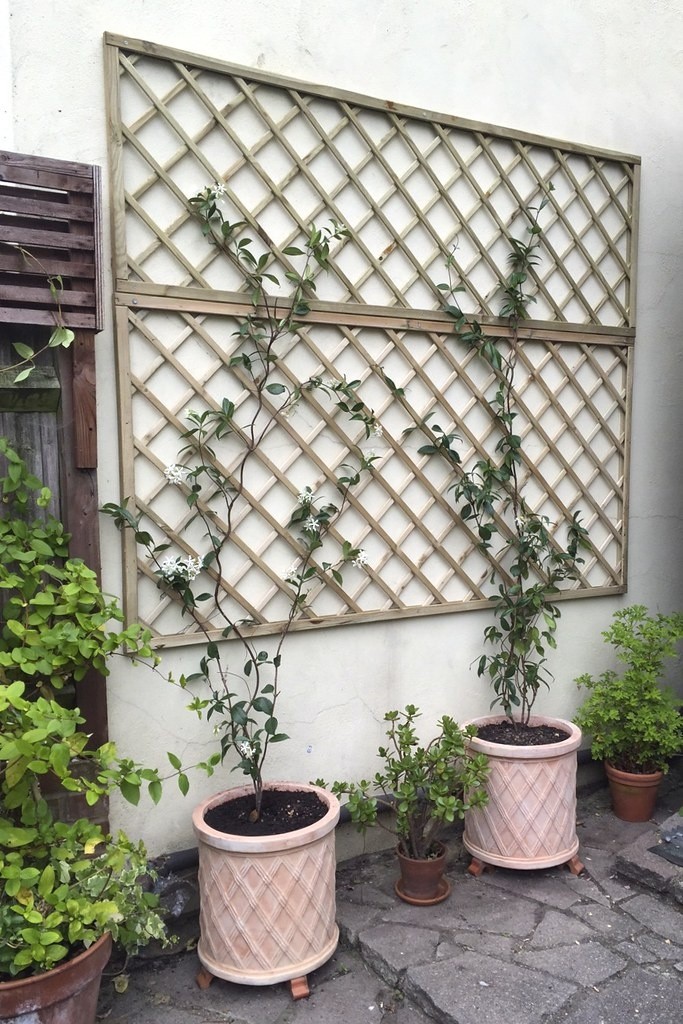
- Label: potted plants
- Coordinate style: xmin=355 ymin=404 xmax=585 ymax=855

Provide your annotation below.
xmin=0 ymin=439 xmax=223 ymax=1024
xmin=570 ymin=602 xmax=683 ymax=823
xmin=307 ymin=704 xmax=494 ymax=907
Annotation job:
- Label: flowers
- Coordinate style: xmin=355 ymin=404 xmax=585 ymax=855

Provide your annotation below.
xmin=97 ymin=179 xmax=385 ymax=824
xmin=401 ymin=173 xmax=587 ymax=729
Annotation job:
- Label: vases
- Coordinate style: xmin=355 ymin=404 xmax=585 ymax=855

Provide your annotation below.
xmin=458 ymin=711 xmax=586 ymax=876
xmin=190 ymin=780 xmax=342 ymax=1000
